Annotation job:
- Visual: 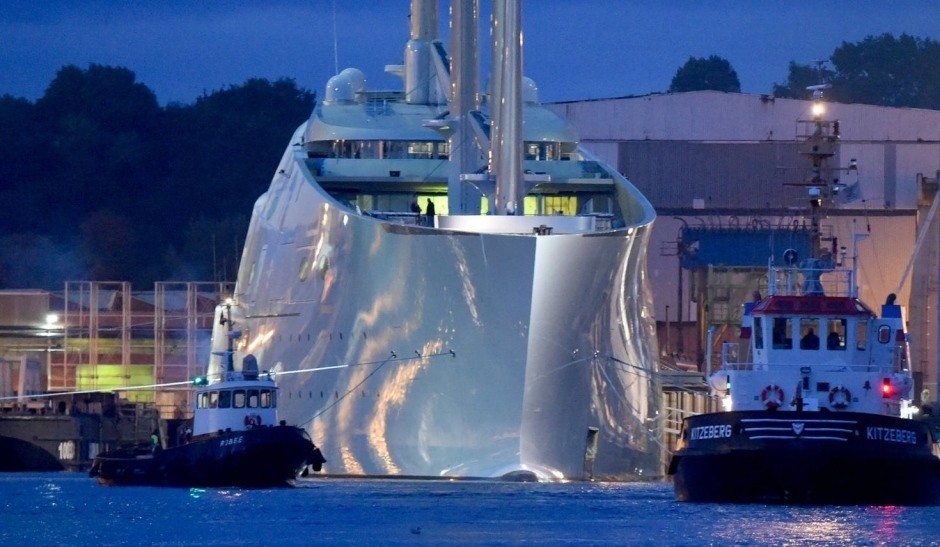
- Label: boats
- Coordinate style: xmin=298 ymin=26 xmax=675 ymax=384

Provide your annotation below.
xmin=667 ymin=57 xmax=940 ymax=508
xmin=88 ymin=304 xmax=327 ymax=488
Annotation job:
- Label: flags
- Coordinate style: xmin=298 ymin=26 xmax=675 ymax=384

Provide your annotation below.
xmin=834 ymin=181 xmax=864 ymax=205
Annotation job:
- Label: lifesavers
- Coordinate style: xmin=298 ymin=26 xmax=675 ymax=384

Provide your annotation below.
xmin=828 ymin=385 xmax=851 ymax=408
xmin=243 ymin=413 xmax=261 ymax=430
xmin=761 ymin=384 xmax=786 ymax=407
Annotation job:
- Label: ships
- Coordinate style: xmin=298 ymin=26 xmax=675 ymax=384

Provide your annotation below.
xmin=201 ymin=2 xmax=667 ymax=484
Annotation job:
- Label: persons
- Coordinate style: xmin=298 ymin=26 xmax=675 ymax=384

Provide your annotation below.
xmin=425 ymin=197 xmax=436 ymax=227
xmin=799 ymin=327 xmax=821 ymax=351
xmin=410 ymin=197 xmax=422 ymax=216
